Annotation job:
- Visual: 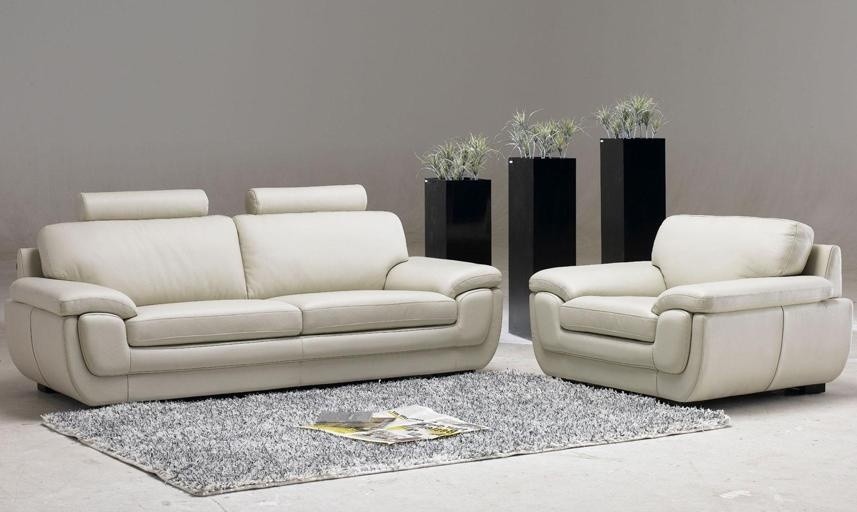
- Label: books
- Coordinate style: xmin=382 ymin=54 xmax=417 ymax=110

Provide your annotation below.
xmin=313 ymin=410 xmax=374 ymax=428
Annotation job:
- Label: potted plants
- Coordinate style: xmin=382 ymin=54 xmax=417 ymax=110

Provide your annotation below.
xmin=508 ymin=108 xmax=583 ymax=340
xmin=596 ymin=99 xmax=667 ymax=264
xmin=423 ymin=132 xmax=493 ymax=268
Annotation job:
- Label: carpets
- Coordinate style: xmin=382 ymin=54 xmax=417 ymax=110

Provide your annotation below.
xmin=37 ymin=370 xmax=732 ymax=496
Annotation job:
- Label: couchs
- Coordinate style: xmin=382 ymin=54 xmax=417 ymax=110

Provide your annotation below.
xmin=7 ymin=183 xmax=506 ymax=408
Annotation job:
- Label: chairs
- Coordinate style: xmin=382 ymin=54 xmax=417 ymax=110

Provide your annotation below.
xmin=523 ymin=211 xmax=854 ymax=408
